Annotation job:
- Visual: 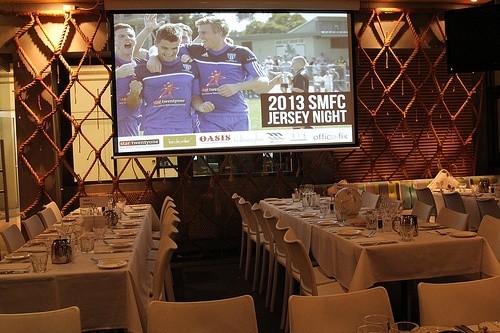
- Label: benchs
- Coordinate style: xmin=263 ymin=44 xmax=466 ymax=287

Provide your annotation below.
xmin=323 ymin=175 xmax=500 ymax=210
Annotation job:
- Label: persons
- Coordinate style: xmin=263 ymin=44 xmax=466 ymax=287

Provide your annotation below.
xmin=114 ymin=16 xmax=269 ymax=137
xmin=268 ymin=54 xmax=344 ymax=93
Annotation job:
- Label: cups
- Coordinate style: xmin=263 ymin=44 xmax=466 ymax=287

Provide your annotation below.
xmin=311 ymin=194 xmax=320 ymax=210
xmin=459 ymin=183 xmax=466 ymax=193
xmin=29 ymin=252 xmax=48 ymax=273
xmin=398 ymin=222 xmax=414 ymax=242
xmin=320 ymin=200 xmax=330 ymax=214
xmin=81 ymin=237 xmax=94 ymax=254
xmin=92 ymin=225 xmax=107 ymax=242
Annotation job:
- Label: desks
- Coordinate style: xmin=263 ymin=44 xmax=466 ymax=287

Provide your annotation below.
xmin=432 ymin=189 xmax=500 ymax=226
xmin=259 ymin=198 xmax=500 ymax=317
xmin=0 ymin=204 xmax=160 ymax=333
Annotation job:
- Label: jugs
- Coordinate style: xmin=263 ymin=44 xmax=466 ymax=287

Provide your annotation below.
xmin=52 ymin=238 xmax=73 ymax=264
xmin=392 ymin=215 xmax=417 ymax=237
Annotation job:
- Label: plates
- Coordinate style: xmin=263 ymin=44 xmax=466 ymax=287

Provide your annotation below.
xmin=337 ymin=229 xmax=362 ymax=236
xmin=122 ymin=206 xmax=148 ymax=225
xmin=449 ymin=231 xmax=477 ymax=238
xmin=97 ymin=259 xmax=127 ymax=268
xmin=5 ymin=252 xmax=30 ymax=260
xmin=300 ymin=214 xmax=320 ymax=218
xmin=31 ymin=239 xmax=47 ymax=244
xmin=108 ymin=242 xmax=135 ymax=248
xmin=317 ymin=221 xmax=337 ymax=225
xmin=115 ymin=231 xmax=138 ymax=236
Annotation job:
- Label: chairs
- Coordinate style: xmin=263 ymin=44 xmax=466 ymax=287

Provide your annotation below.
xmin=0 ymin=169 xmax=500 ymax=333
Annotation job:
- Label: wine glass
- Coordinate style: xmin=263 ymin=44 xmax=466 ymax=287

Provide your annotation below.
xmin=71 ymin=221 xmax=85 ymax=250
xmin=304 ymin=185 xmax=314 ymax=207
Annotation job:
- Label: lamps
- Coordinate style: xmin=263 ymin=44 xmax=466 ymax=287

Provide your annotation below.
xmin=63 ymin=5 xmax=75 ymax=12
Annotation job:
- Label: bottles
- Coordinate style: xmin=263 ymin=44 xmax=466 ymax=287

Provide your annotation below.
xmin=378 ymin=218 xmax=383 ymax=232
xmin=385 ymin=217 xmax=392 ymax=232
